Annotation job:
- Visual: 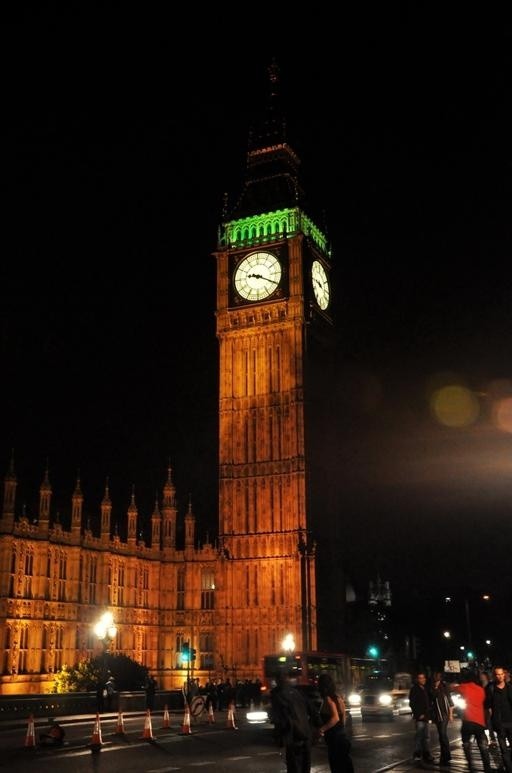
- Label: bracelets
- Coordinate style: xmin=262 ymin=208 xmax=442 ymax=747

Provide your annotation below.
xmin=320 ymin=728 xmax=325 ymax=733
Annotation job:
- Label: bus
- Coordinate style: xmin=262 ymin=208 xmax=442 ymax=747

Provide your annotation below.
xmin=258 ymin=651 xmax=387 ymax=696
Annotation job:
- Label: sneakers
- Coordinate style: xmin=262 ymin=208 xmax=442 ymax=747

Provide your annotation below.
xmin=414 ymin=753 xmax=435 ymax=762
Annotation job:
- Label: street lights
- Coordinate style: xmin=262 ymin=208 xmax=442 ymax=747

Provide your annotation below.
xmin=94 ymin=610 xmax=118 ymax=665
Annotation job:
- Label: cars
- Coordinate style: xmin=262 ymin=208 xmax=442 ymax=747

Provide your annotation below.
xmin=345 ymin=672 xmax=467 ymax=721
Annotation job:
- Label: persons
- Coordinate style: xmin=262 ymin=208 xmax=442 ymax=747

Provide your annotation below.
xmin=315 ymin=673 xmax=354 ymax=772
xmin=96 ymin=674 xmax=263 ymax=714
xmin=275 ymin=684 xmax=313 ymax=772
xmin=407 ymin=662 xmax=511 ymax=771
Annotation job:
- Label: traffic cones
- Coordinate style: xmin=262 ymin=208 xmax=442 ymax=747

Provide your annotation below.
xmin=23 ymin=702 xmax=239 ymax=750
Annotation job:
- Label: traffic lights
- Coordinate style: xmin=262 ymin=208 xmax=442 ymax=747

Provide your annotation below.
xmin=183 ymin=643 xmax=189 ymax=662
xmin=190 ymin=648 xmax=196 ymax=660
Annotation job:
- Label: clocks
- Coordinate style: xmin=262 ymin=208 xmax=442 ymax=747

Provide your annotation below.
xmin=308 ymin=257 xmax=332 ymax=311
xmin=232 ymin=249 xmax=286 ymax=303
